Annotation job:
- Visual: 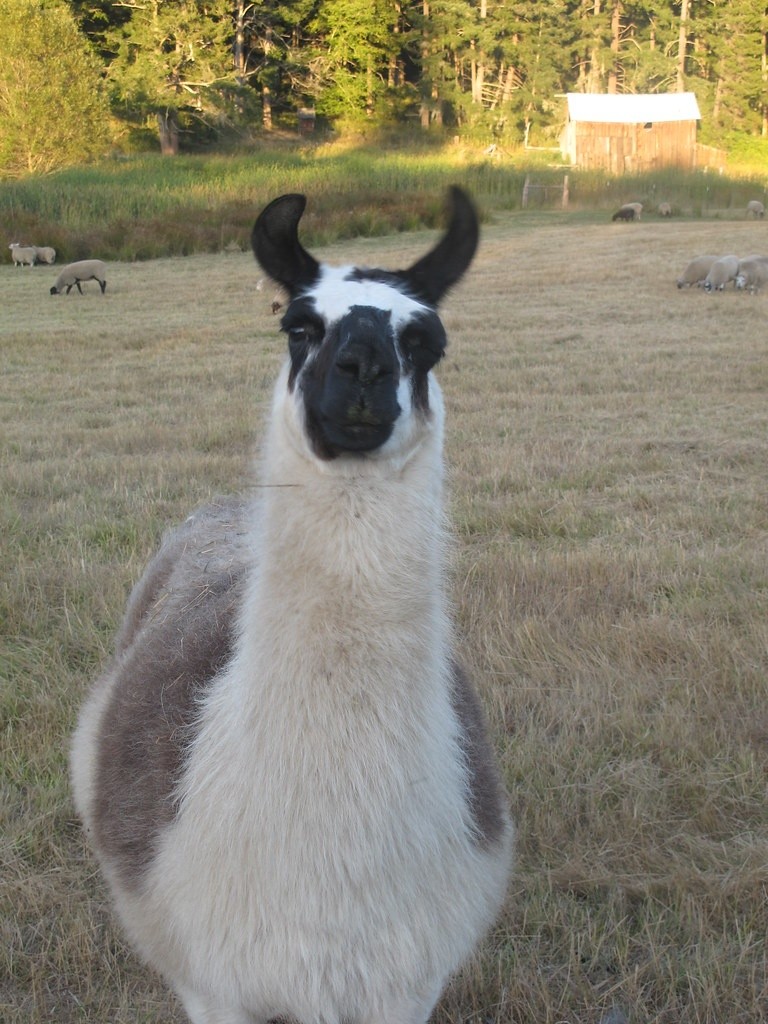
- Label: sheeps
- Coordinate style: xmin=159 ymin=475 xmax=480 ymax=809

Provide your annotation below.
xmin=271 ymin=293 xmax=286 ymax=314
xmin=50 ymin=259 xmax=107 ymax=296
xmin=675 ymin=255 xmax=768 ymax=295
xmin=9 ymin=242 xmax=55 ymax=267
xmin=658 ymin=202 xmax=673 ymax=217
xmin=746 ymin=201 xmax=765 ymax=218
